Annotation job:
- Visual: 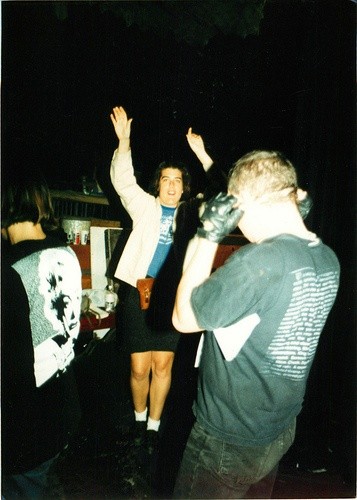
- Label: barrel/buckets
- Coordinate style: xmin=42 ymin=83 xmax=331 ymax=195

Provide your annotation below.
xmin=63 ymin=219 xmax=92 ymax=240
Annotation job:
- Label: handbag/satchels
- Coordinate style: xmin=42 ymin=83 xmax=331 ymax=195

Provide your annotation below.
xmin=137 ymin=278 xmax=155 ymax=309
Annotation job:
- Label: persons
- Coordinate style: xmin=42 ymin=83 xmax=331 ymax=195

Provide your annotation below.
xmin=171 ymin=150 xmax=341 ymax=498
xmin=109 ymin=105 xmax=225 ymax=455
xmin=1 ymin=166 xmax=83 ymax=500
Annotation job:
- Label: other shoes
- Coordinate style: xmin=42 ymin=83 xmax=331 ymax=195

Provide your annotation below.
xmin=144 ymin=429 xmax=160 ymax=449
xmin=130 ymin=421 xmax=147 ymax=446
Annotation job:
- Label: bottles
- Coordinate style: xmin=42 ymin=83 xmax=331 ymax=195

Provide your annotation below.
xmin=67 ymin=230 xmax=89 ymax=245
xmin=104 ymin=286 xmax=115 ymax=312
